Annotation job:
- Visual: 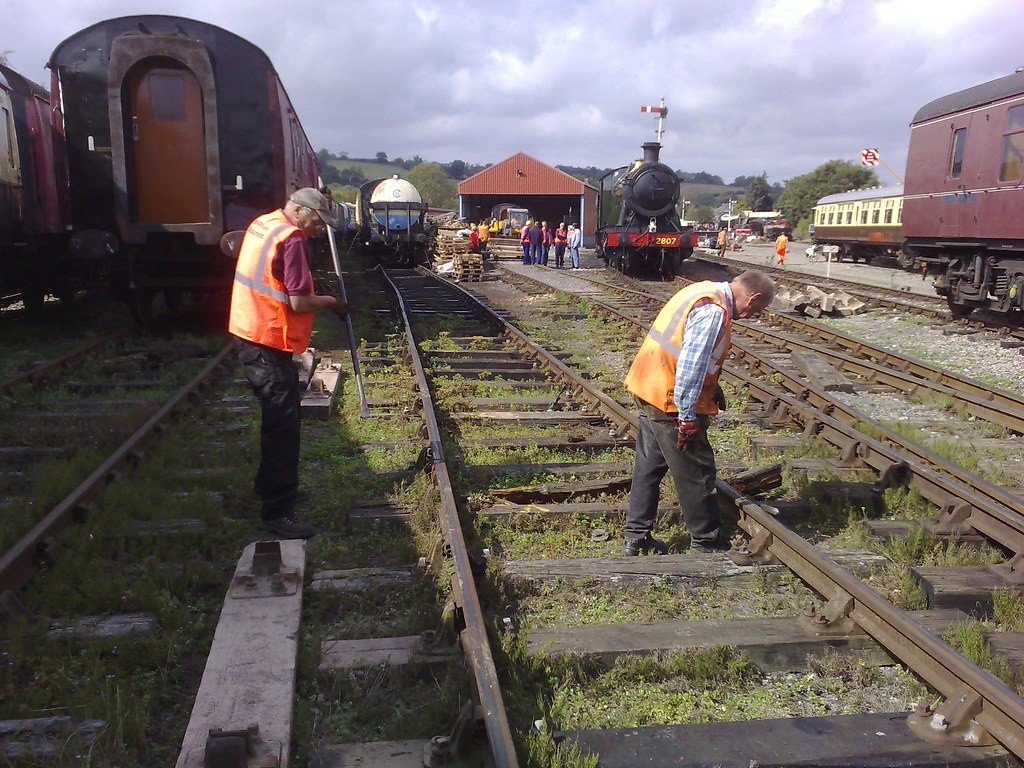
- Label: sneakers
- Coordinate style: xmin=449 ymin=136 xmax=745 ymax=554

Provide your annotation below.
xmin=262 ymin=511 xmax=317 ymax=540
xmin=253 ymin=486 xmax=311 ymax=500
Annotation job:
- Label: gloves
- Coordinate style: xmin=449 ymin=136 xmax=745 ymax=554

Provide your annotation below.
xmin=677 ymin=419 xmax=709 ymax=452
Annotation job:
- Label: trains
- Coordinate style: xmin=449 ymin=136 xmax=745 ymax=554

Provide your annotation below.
xmin=808 ymin=186 xmax=913 ymax=267
xmin=42 ymin=15 xmax=354 ymax=326
xmin=0 ymin=66 xmax=79 ymax=309
xmin=594 ymin=97 xmax=698 ymax=283
xmin=355 ymin=171 xmax=435 ymax=256
xmin=901 ymin=67 xmax=1024 ymax=322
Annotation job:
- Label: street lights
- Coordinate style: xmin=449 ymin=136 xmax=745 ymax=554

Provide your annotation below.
xmin=728 ymin=197 xmax=738 ymax=231
xmin=682 ymin=197 xmax=691 ymax=222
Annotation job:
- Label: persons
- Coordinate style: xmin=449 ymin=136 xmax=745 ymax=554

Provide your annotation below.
xmin=479 ymin=220 xmax=494 ymax=250
xmin=717 ymin=227 xmax=730 ymax=257
xmin=519 ymin=220 xmax=532 ymax=265
xmin=529 ymin=221 xmax=544 ymax=266
xmin=624 ymin=269 xmax=774 ymax=554
xmin=470 ymin=226 xmax=479 ymax=247
xmin=775 ymin=231 xmax=788 ymax=265
xmin=554 ymin=222 xmax=567 ymax=269
xmin=228 ymin=187 xmax=351 ymax=539
xmin=569 ymin=222 xmax=580 ymax=269
xmin=541 ymin=221 xmax=552 ymax=266
xmin=805 ymin=242 xmax=817 ymax=261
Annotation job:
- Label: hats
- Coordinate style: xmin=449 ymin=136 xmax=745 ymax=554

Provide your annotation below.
xmin=289 ymin=187 xmax=339 ymax=229
xmin=472 ymin=226 xmax=478 ymax=230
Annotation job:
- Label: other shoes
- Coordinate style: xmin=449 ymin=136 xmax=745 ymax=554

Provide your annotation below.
xmin=623 ymin=536 xmax=666 ymax=556
xmin=523 ymin=262 xmax=579 ymax=270
xmin=689 ymin=541 xmax=736 ymax=556
